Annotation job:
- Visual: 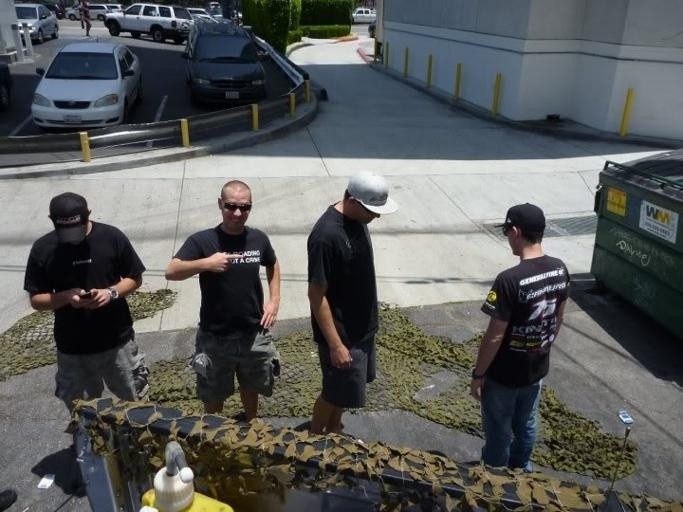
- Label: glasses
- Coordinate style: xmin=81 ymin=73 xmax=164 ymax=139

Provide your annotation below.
xmin=502 ymin=227 xmax=508 ymax=236
xmin=224 ymin=202 xmax=251 ymax=211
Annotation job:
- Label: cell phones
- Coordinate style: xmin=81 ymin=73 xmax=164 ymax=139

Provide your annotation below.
xmin=80 ymin=291 xmax=91 ymax=299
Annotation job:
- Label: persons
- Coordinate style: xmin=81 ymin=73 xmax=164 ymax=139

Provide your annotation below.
xmin=166 ymin=181 xmax=280 ymax=418
xmin=309 ymin=174 xmax=398 ymax=432
xmin=80 ymin=0 xmax=92 ymax=37
xmin=472 ymin=203 xmax=569 ymax=466
xmin=23 ymin=193 xmax=150 ymax=433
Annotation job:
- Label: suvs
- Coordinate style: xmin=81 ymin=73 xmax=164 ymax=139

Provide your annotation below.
xmin=65 ymin=3 xmax=109 ymax=20
xmin=105 ymin=3 xmax=194 ymax=44
xmin=185 ymin=21 xmax=266 ymax=102
xmin=105 ymin=3 xmax=123 ymax=13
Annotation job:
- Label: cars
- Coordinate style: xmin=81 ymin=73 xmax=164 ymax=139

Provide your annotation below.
xmin=30 ymin=42 xmax=141 ymax=126
xmin=13 ymin=3 xmax=60 ymax=42
xmin=187 ymin=2 xmax=242 ymax=20
xmin=0 ymin=60 xmax=13 ymax=115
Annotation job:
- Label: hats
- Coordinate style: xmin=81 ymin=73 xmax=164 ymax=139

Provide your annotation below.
xmin=348 ymin=172 xmax=398 ymax=214
xmin=50 ymin=192 xmax=90 ymax=243
xmin=495 ymin=203 xmax=545 ymax=228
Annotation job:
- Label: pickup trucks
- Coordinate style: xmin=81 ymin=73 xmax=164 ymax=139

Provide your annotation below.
xmin=351 ymin=7 xmax=377 ymax=23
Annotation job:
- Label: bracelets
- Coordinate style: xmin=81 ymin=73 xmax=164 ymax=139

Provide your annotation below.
xmin=472 ymin=369 xmax=485 ymax=380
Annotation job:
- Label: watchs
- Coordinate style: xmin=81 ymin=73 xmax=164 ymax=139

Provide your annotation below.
xmin=108 ymin=286 xmax=119 ymax=301
